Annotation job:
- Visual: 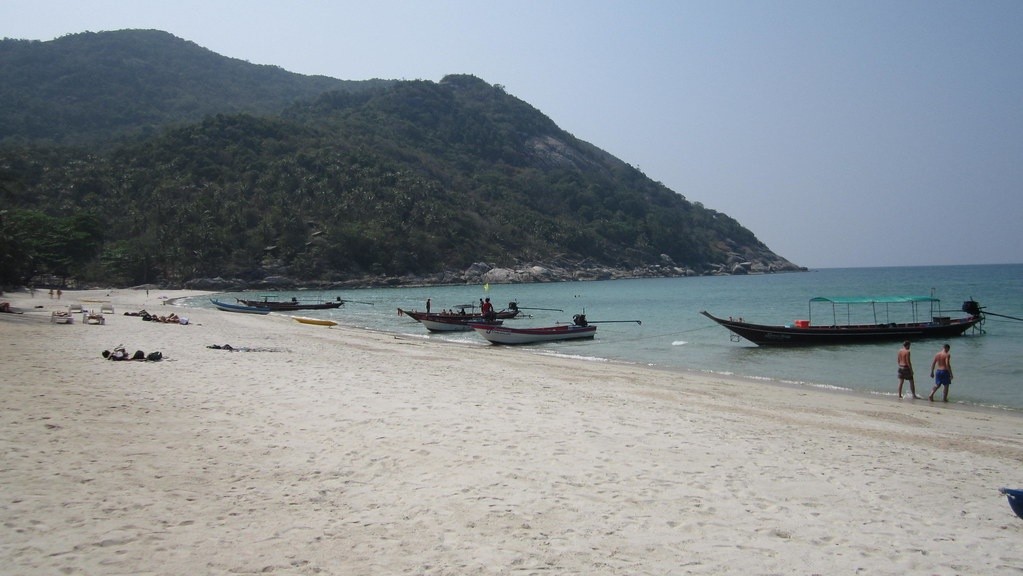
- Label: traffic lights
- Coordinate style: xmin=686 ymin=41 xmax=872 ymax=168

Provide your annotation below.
xmin=208 ymin=298 xmax=271 ymax=315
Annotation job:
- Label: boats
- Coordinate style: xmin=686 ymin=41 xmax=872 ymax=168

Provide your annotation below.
xmin=291 ymin=316 xmax=339 ymax=327
xmin=396 ymin=296 xmax=521 ymax=332
xmin=236 ymin=294 xmax=345 ymax=313
xmin=465 ymin=309 xmax=598 ymax=347
xmin=701 ymin=294 xmax=990 ymax=347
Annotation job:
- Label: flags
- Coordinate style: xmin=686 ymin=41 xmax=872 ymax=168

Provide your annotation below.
xmin=482 ymin=281 xmax=490 ymax=292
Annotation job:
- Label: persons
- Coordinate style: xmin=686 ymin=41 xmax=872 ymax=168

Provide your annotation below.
xmin=425 ymin=298 xmax=431 ymax=313
xmin=929 ymin=344 xmax=953 ymax=403
xmin=441 ymin=309 xmax=446 ymax=316
xmin=897 ymin=341 xmax=921 ymax=400
xmin=483 ymin=297 xmax=493 ymax=322
xmin=478 ymin=298 xmax=484 ymax=315
xmin=152 ymin=313 xmax=180 ymax=323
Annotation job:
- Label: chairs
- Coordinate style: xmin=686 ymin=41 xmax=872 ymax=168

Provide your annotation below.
xmin=69 ymin=303 xmax=83 ymax=313
xmin=99 ymin=302 xmax=114 ymax=314
